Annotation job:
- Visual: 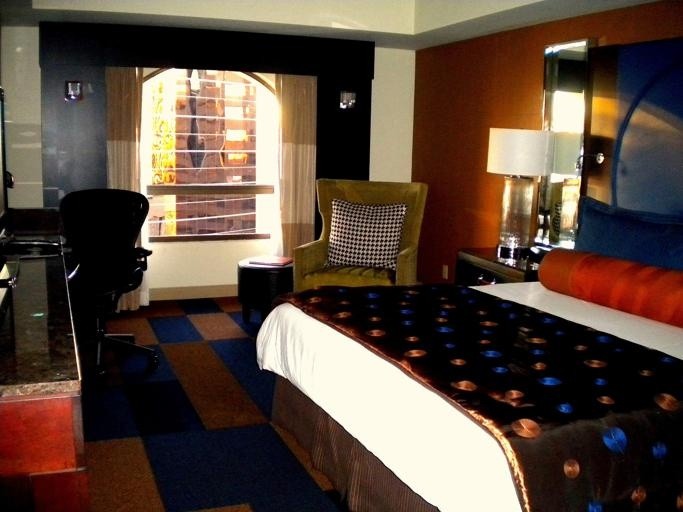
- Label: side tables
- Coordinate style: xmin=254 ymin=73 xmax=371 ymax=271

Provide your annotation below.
xmin=237 ymin=257 xmax=293 ymax=323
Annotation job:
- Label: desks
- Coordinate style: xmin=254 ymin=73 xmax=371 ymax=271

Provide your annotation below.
xmin=0 ymin=209 xmax=90 ymax=512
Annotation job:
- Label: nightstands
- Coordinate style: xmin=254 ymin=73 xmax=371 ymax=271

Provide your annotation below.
xmin=453 ymin=247 xmax=550 ymax=285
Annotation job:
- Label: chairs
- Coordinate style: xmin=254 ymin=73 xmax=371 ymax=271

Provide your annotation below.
xmin=293 ymin=177 xmax=429 ymax=294
xmin=57 ymin=189 xmax=161 ymax=377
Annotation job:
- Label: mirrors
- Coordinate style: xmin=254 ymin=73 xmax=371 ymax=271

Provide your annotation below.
xmin=528 ymin=36 xmax=595 ymax=250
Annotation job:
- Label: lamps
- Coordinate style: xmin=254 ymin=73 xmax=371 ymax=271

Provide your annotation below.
xmin=339 ymin=92 xmax=356 ymax=109
xmin=486 ymin=127 xmax=555 ymax=178
xmin=64 ymin=79 xmax=83 ymax=103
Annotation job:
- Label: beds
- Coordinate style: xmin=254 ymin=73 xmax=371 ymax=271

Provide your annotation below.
xmin=256 ymin=34 xmax=683 ymax=512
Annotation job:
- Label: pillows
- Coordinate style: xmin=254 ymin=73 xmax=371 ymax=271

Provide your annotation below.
xmin=537 ymin=250 xmax=683 ymax=328
xmin=574 ymin=196 xmax=683 ymax=272
xmin=324 ymin=198 xmax=407 ymax=271
